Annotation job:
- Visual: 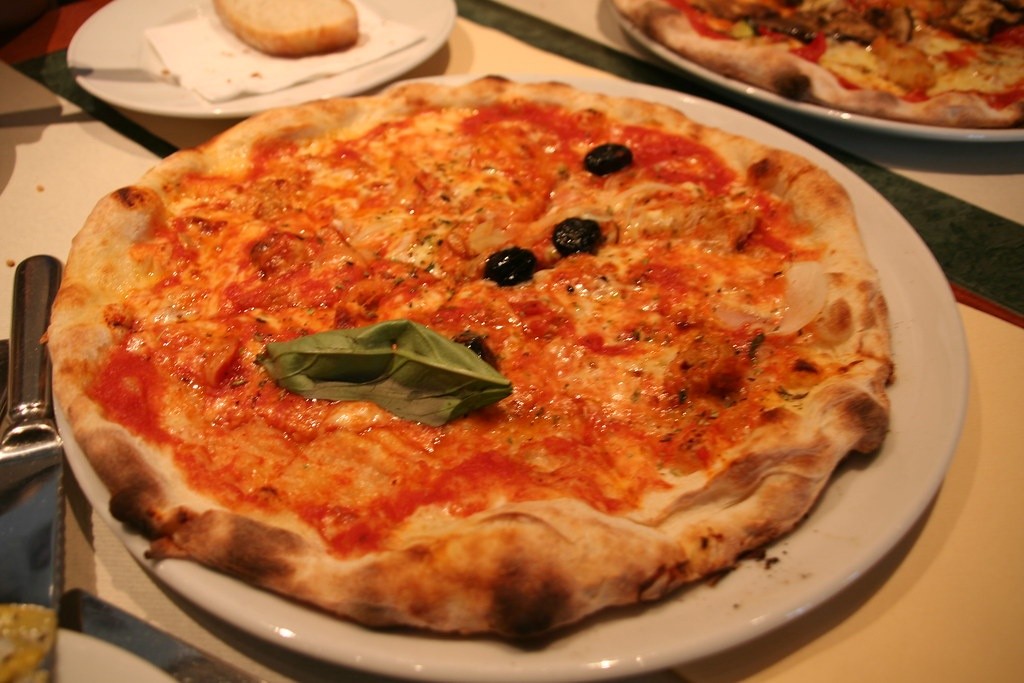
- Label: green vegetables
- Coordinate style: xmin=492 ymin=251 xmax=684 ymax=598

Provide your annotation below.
xmin=263 ymin=321 xmax=512 ymax=426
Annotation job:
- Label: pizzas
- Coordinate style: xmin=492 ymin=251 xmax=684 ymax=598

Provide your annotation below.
xmin=45 ymin=79 xmax=893 ymax=643
xmin=612 ymin=0 xmax=1024 ymax=128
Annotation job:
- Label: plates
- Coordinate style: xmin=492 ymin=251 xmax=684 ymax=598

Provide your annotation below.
xmin=607 ymin=0 xmax=1024 ymax=141
xmin=66 ymin=0 xmax=456 ymax=119
xmin=55 ymin=75 xmax=962 ymax=683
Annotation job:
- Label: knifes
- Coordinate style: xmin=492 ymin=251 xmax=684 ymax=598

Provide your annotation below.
xmin=0 ymin=255 xmax=65 ymax=683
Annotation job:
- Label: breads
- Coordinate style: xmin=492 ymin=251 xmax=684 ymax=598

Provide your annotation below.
xmin=213 ymin=0 xmax=357 ymax=56
